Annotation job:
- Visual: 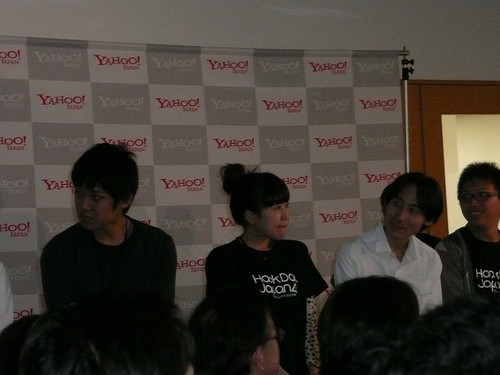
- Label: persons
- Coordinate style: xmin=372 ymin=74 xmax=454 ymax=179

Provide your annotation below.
xmin=40 ymin=143 xmax=178 ymax=350
xmin=436 ymin=161 xmax=500 ymax=303
xmin=204 ymin=163 xmax=328 ymax=374
xmin=317 ymin=275 xmax=500 ymax=374
xmin=334 ymin=172 xmax=445 ymax=315
xmin=0 ymin=286 xmax=284 ymax=374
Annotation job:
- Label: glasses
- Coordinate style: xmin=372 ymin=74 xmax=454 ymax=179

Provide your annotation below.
xmin=458 ymin=191 xmax=499 ymax=203
xmin=261 ymin=329 xmax=285 ymax=345
